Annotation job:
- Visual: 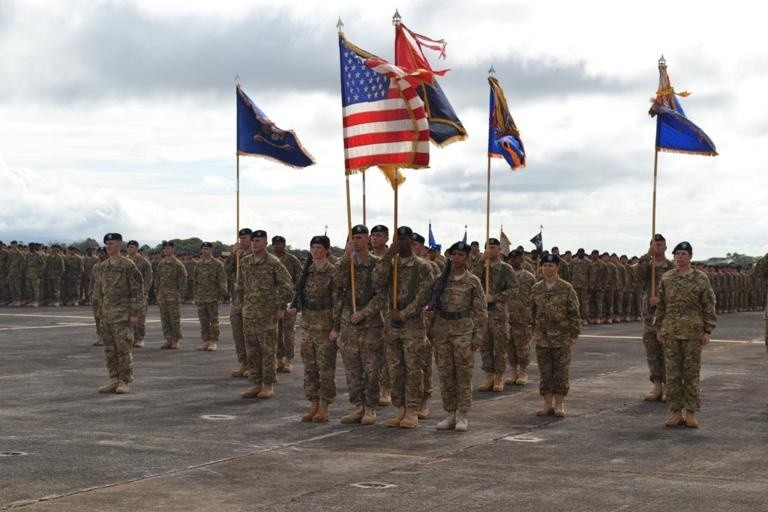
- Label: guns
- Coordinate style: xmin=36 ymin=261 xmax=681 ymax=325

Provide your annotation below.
xmin=426 ymin=259 xmax=452 ymax=311
xmin=290 ymin=254 xmax=312 ymax=310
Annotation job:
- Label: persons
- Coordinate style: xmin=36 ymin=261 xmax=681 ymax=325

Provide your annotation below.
xmin=224 ymin=224 xmax=580 ymax=432
xmin=1 ymin=240 xmax=109 ymax=306
xmin=88 ymin=232 xmax=231 ymax=394
xmin=635 ymin=233 xmax=716 ymax=428
xmin=564 ymin=247 xmax=767 ymax=325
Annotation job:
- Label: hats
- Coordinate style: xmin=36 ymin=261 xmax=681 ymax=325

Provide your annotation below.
xmin=650 ymin=234 xmax=665 ymax=245
xmin=672 ymin=241 xmax=692 ymax=254
xmin=310 ymin=236 xmax=331 ymax=249
xmin=350 ymin=225 xmax=425 ymax=244
xmin=239 ymin=228 xmax=285 ymax=243
xmin=428 ymin=238 xmax=639 ymax=265
xmin=0 ymin=232 xmax=214 ymax=251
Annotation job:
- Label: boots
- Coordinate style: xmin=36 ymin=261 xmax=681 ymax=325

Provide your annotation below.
xmin=478 ymin=364 xmax=529 ymax=393
xmin=303 ymin=385 xmax=470 ymax=432
xmin=257 ymin=381 xmax=274 ymax=398
xmin=536 ymin=392 xmax=553 ymax=415
xmin=241 ymin=382 xmax=261 ymax=398
xmin=645 ymin=379 xmax=699 ymax=428
xmin=94 ymin=337 xmax=217 ymax=351
xmin=553 ymin=392 xmax=565 ymax=417
xmin=582 ymin=306 xmax=763 ymax=325
xmin=99 ymin=376 xmax=119 ymax=393
xmin=0 ymin=296 xmax=233 ymax=307
xmin=116 ymin=379 xmax=129 ymax=393
xmin=231 ymin=356 xmax=292 ymax=377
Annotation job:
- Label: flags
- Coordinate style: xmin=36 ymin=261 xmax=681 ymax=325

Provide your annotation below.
xmin=337 ymin=31 xmax=431 ymax=190
xmin=395 ymin=22 xmax=470 ymax=150
xmin=235 ymin=83 xmax=316 ymax=169
xmin=487 ymin=76 xmax=527 ymax=171
xmin=648 ymin=53 xmax=720 ymax=157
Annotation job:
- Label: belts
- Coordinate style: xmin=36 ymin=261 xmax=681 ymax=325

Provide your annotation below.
xmin=302 ymin=302 xmax=333 ymax=310
xmin=439 ymin=310 xmax=471 ymax=319
